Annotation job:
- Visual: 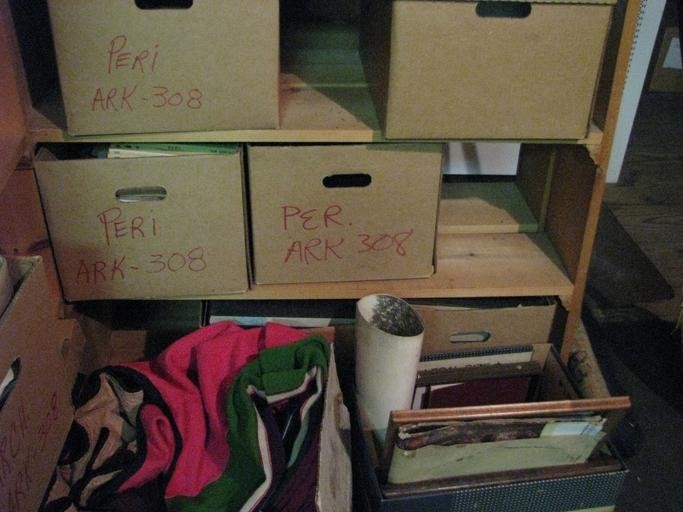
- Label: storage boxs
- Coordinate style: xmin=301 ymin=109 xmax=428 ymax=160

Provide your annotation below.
xmin=29 ymin=148 xmax=250 ymax=302
xmin=198 ymin=303 xmax=355 ymax=359
xmin=47 ymin=1 xmax=278 ymax=135
xmin=247 ymin=144 xmax=447 ymax=284
xmin=360 ymin=0 xmax=620 ymax=141
xmin=0 ymin=255 xmax=75 ymax=512
xmin=413 ymin=298 xmax=558 ymax=356
xmin=345 ymin=344 xmax=628 ymax=512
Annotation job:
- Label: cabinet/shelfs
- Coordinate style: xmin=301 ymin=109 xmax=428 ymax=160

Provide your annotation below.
xmin=0 ymin=1 xmax=639 ymax=368
